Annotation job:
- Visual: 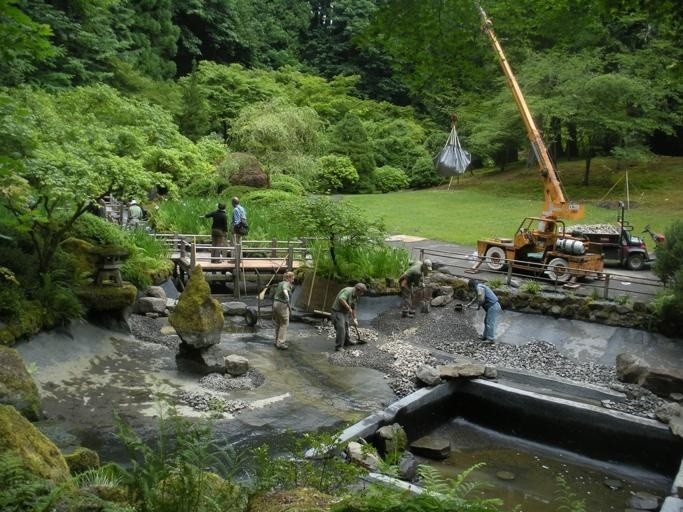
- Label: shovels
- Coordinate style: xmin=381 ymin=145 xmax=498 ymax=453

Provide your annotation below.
xmin=454 ymin=304 xmax=479 ymax=312
xmin=420 ymin=271 xmax=429 ymax=314
xmin=352 ymin=314 xmax=367 ymax=344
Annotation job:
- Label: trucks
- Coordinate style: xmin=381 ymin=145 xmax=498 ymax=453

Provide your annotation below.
xmin=557 ymin=199 xmax=665 ymax=270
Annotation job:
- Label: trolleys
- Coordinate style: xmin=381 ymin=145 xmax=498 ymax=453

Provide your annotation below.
xmin=245 ymin=263 xmax=280 ymax=326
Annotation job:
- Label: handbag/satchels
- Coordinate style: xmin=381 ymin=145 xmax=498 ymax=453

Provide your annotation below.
xmin=234 ymin=221 xmax=249 ymax=235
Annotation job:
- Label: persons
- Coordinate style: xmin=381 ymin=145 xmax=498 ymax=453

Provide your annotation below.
xmin=329 ymin=282 xmax=368 ymax=352
xmin=463 ymin=276 xmax=501 ymax=345
xmin=197 ymin=203 xmax=228 ymax=264
xmin=126 ymin=199 xmax=142 ymax=232
xmin=271 ymin=270 xmax=296 ymax=349
xmin=397 ymin=257 xmax=432 ymax=319
xmin=228 ymin=197 xmax=248 ymax=265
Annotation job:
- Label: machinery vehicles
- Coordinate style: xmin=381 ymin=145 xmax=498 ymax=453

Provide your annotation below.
xmin=477 ymin=5 xmax=607 ymax=287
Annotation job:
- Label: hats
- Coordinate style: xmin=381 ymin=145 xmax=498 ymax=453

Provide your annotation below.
xmin=131 ymin=199 xmax=137 ymax=204
xmin=424 ymin=259 xmax=433 ymax=270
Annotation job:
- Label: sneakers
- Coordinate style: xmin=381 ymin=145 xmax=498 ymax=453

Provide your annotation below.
xmin=479 ymin=336 xmax=494 ymax=344
xmin=278 ymin=344 xmax=288 ymax=350
xmin=349 ymin=342 xmax=356 ymax=345
xmin=336 ymin=348 xmax=344 ymax=351
xmin=402 ymin=310 xmax=416 ymax=317
xmin=228 ymin=260 xmax=235 ymax=263
